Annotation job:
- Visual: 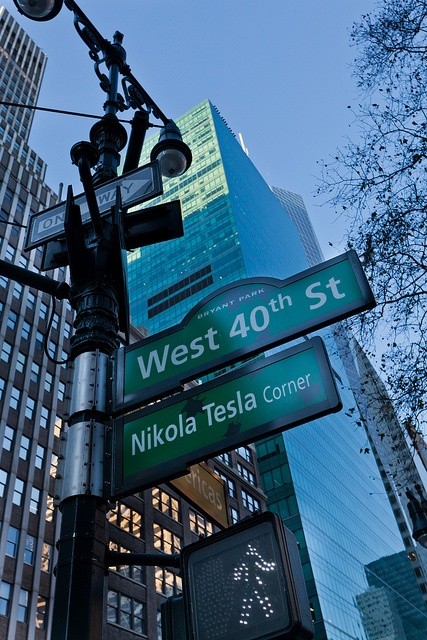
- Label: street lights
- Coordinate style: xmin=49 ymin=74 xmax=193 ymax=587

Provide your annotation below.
xmin=12 ymin=0 xmax=192 ymax=640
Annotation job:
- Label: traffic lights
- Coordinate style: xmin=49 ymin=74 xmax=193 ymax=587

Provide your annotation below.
xmin=180 ymin=512 xmax=314 ymax=640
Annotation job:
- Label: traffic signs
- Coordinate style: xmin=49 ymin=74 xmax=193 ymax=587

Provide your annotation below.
xmin=111 ymin=336 xmax=341 ymax=499
xmin=112 ymin=252 xmax=375 ymax=415
xmin=23 ymin=161 xmax=163 ymax=252
